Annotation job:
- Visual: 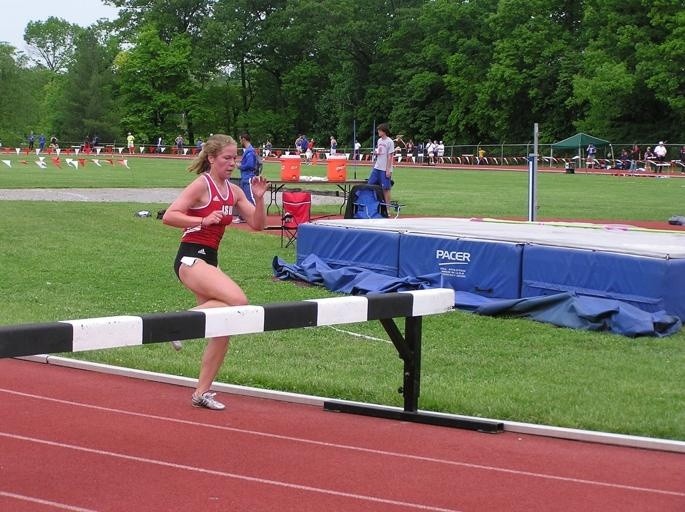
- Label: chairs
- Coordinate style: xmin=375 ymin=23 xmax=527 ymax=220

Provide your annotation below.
xmin=344 ymin=184 xmax=405 ymax=219
xmin=281 ymin=192 xmax=312 ymax=249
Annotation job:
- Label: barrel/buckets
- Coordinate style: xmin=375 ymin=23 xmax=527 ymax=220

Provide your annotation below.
xmin=326 ymin=156 xmax=345 ymax=181
xmin=280 ymin=155 xmax=301 ymax=181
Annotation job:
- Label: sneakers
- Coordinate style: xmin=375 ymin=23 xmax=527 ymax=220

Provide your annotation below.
xmin=232 ymin=217 xmax=245 ymax=224
xmin=192 ymin=390 xmax=225 ymax=410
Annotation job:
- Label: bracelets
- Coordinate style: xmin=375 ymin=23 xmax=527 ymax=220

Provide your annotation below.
xmin=200 ymin=215 xmax=204 ymax=227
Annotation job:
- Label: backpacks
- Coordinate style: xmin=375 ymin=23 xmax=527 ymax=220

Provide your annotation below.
xmin=246 ymin=152 xmax=263 ymax=176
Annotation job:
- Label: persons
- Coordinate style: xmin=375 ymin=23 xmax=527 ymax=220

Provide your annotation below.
xmin=231 ymin=133 xmax=259 ymax=224
xmin=330 ymin=136 xmax=337 ymax=154
xmin=28 ymin=130 xmax=59 ymax=152
xmin=175 ymin=134 xmax=183 ymax=155
xmin=197 ymin=137 xmax=203 ymax=149
xmin=78 ymin=135 xmax=98 ymax=154
xmin=127 ymin=132 xmax=135 ymax=155
xmin=368 ymin=124 xmax=395 ymax=217
xmin=264 ymin=138 xmax=272 ymax=158
xmin=478 ymin=147 xmax=486 ymax=165
xmin=586 ymin=141 xmax=668 ymax=173
xmin=355 ymin=140 xmax=362 ymax=154
xmin=164 ymin=136 xmax=272 ymax=411
xmin=294 ymin=133 xmax=315 ymax=167
xmin=392 ymin=135 xmax=445 ymax=165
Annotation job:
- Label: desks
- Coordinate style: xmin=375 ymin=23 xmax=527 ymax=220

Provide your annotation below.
xmin=231 ymin=177 xmax=368 ymax=216
